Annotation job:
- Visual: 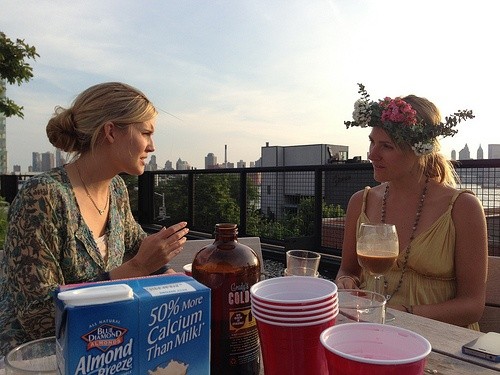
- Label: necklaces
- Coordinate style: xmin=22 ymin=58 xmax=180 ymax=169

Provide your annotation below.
xmin=380 ymin=178 xmax=428 ymax=302
xmin=74 ymin=160 xmax=109 ymax=215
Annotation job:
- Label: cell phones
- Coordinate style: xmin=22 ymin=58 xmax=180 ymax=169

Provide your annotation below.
xmin=461 ymin=331 xmax=500 ymax=363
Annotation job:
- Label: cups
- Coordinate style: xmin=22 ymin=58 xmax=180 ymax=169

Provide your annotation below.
xmin=178 ymin=261 xmax=192 ymax=276
xmin=249 ymin=249 xmax=432 ymax=375
xmin=3 ymin=336 xmax=61 ymax=375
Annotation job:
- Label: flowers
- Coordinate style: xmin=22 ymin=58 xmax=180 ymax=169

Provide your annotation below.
xmin=344 ymin=82 xmax=475 ymax=156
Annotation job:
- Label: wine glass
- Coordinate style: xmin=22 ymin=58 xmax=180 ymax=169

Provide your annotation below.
xmin=356 ymin=222 xmax=399 ymax=322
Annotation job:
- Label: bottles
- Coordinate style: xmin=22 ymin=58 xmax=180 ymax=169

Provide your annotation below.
xmin=192 ymin=222 xmax=261 ymax=375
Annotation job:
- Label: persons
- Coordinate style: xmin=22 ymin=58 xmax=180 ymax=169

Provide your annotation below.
xmin=336 ymin=83 xmax=488 ymax=332
xmin=0 ymin=81 xmax=189 ymax=361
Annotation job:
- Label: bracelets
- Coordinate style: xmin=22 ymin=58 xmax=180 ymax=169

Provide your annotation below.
xmin=337 ymin=276 xmax=359 ymax=286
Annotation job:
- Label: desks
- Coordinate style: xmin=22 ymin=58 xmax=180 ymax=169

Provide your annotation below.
xmin=0 ymin=290 xmax=500 ymax=375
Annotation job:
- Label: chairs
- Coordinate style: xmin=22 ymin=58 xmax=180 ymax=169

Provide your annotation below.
xmin=479 ymin=257 xmax=500 ymax=333
xmin=168 ymin=237 xmax=265 ymax=280
